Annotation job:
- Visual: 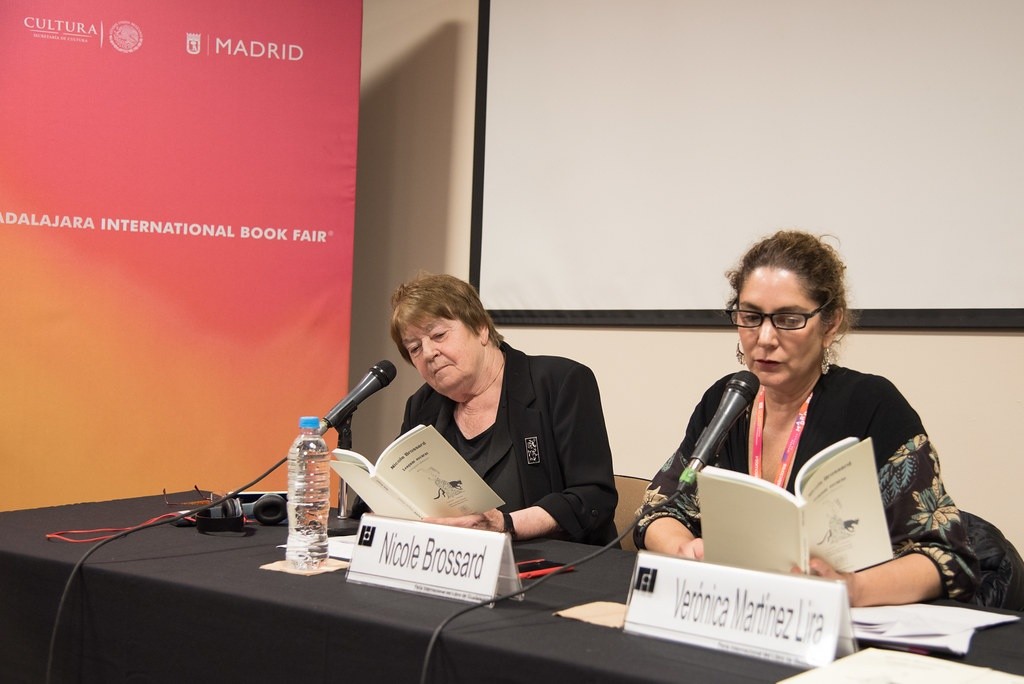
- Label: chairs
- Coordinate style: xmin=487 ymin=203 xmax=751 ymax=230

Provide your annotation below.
xmin=612 ymin=473 xmax=655 ymax=553
xmin=954 ymin=508 xmax=1024 ymax=616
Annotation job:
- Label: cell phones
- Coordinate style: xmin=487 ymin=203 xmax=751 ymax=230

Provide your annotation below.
xmin=515 ymin=559 xmax=574 ymax=580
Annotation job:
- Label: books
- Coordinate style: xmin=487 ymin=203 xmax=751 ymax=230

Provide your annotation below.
xmin=696 ymin=435 xmax=896 ymax=582
xmin=327 ymin=424 xmax=506 ymax=523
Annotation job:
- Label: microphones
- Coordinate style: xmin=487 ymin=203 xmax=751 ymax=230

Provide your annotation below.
xmin=677 ymin=371 xmax=760 ymax=494
xmin=319 ymin=360 xmax=397 ymax=436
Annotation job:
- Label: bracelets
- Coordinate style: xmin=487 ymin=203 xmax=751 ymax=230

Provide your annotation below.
xmin=501 ymin=513 xmax=516 ymax=539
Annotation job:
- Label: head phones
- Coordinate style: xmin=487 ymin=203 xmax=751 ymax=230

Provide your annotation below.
xmin=194 ymin=493 xmax=287 ymax=533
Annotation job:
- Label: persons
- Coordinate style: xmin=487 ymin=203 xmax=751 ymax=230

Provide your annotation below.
xmin=349 ymin=272 xmax=622 ymax=550
xmin=632 ymin=228 xmax=981 ymax=612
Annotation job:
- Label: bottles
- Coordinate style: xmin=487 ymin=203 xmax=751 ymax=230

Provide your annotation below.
xmin=286 ymin=415 xmax=331 ymax=572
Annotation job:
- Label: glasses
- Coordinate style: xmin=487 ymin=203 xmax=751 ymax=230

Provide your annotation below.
xmin=725 ymin=294 xmax=834 ymax=330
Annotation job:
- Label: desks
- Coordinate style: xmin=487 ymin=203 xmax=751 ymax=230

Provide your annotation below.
xmin=0 ymin=489 xmax=1024 ymax=684
